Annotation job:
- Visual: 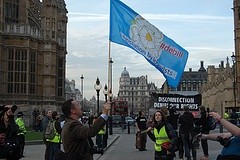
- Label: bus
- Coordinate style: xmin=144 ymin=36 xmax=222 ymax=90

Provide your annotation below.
xmin=103 ymin=100 xmax=129 ymax=117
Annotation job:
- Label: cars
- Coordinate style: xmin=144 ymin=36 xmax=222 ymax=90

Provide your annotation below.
xmin=112 ymin=115 xmax=134 ymax=127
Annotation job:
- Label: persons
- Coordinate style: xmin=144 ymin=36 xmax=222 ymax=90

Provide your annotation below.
xmin=61 ymin=99 xmax=111 ymax=160
xmin=0 ymin=105 xmax=27 ymax=160
xmin=197 ymin=111 xmax=240 ymax=160
xmin=88 ymin=113 xmax=93 ymax=126
xmin=32 ymin=108 xmax=65 ymax=160
xmin=95 ymin=112 xmax=106 ymax=147
xmin=136 ymin=106 xmax=211 ymax=160
xmin=224 ymin=109 xmax=239 ymax=126
xmin=120 ymin=112 xmax=126 ymax=133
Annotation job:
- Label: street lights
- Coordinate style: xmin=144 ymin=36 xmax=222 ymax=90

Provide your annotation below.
xmin=108 ymin=88 xmax=112 ymax=135
xmin=94 ymin=76 xmax=102 ymax=145
xmin=103 ymin=84 xmax=108 ymax=139
xmin=231 ymin=54 xmax=236 ymax=112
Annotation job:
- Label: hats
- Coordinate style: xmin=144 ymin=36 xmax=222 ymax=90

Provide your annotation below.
xmin=17 ymin=112 xmax=24 ymax=117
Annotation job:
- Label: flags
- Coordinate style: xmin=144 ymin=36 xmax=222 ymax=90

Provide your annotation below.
xmin=110 ymin=0 xmax=189 ymax=88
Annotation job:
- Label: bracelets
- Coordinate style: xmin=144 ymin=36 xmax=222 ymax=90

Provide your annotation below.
xmin=217 ymin=116 xmax=223 ymax=122
xmin=205 ymin=135 xmax=207 ymax=140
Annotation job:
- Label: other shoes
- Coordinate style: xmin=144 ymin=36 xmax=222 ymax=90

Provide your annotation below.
xmin=139 ymin=148 xmax=147 ymax=151
xmin=200 ymin=155 xmax=208 ymax=160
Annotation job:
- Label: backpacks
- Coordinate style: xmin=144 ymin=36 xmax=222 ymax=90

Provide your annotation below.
xmin=44 ymin=121 xmax=58 ymax=140
